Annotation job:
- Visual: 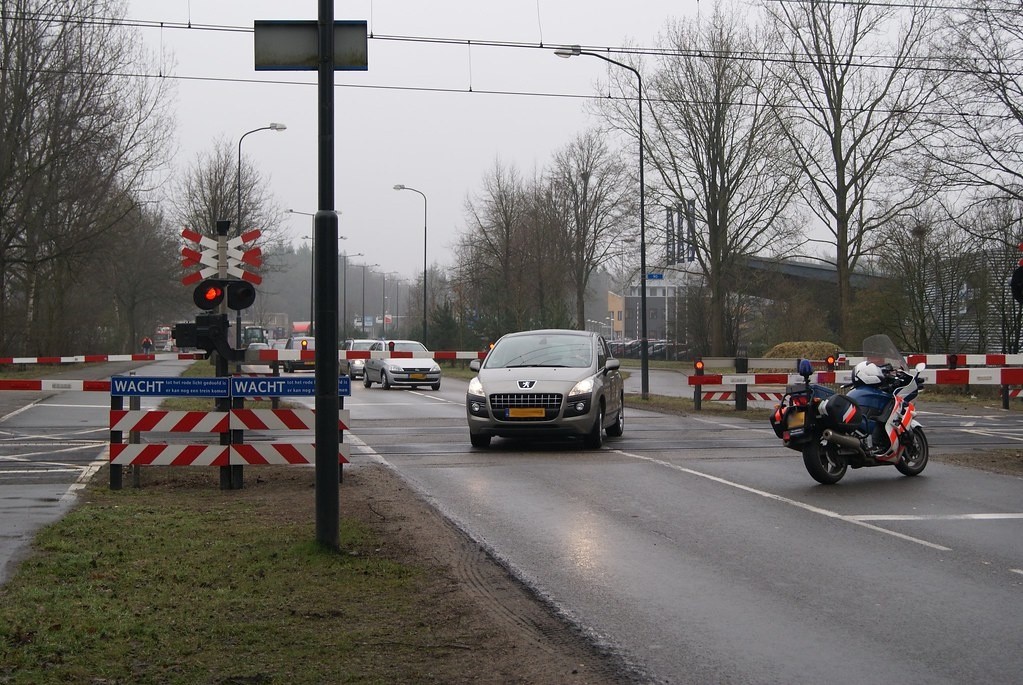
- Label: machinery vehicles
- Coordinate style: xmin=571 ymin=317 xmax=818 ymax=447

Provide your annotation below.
xmin=241 ymin=325 xmax=269 ymax=353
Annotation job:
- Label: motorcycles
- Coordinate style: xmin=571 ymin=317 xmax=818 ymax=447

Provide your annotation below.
xmin=770 ymin=355 xmax=929 ymax=485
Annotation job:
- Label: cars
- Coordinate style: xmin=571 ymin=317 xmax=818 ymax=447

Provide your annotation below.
xmin=604 ymin=338 xmax=692 ymax=361
xmin=466 ymin=328 xmax=626 ymax=449
xmin=361 ymin=340 xmax=442 ymax=390
xmin=246 ymin=338 xmax=289 ymax=367
xmin=338 ymin=336 xmax=380 ymax=379
xmin=283 ymin=336 xmax=315 ymax=372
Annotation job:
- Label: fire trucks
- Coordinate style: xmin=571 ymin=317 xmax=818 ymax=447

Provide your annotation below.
xmin=155 ymin=328 xmax=175 ymax=348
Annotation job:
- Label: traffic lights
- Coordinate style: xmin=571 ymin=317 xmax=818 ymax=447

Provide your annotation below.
xmin=194 ymin=279 xmax=225 ymax=311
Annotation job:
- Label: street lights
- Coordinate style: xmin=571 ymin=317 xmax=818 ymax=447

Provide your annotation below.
xmin=392 ymin=184 xmax=427 ymax=344
xmin=620 ymin=239 xmax=635 ymax=293
xmin=287 ymin=208 xmax=409 ymax=342
xmin=586 ymin=317 xmax=614 ymax=339
xmin=555 ymin=45 xmax=650 ymax=399
xmin=238 ymin=122 xmax=288 ymax=373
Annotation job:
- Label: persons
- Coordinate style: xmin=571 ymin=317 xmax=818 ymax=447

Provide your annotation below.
xmin=250 ymin=331 xmax=256 ymax=337
xmin=142 ymin=336 xmax=152 ymax=354
